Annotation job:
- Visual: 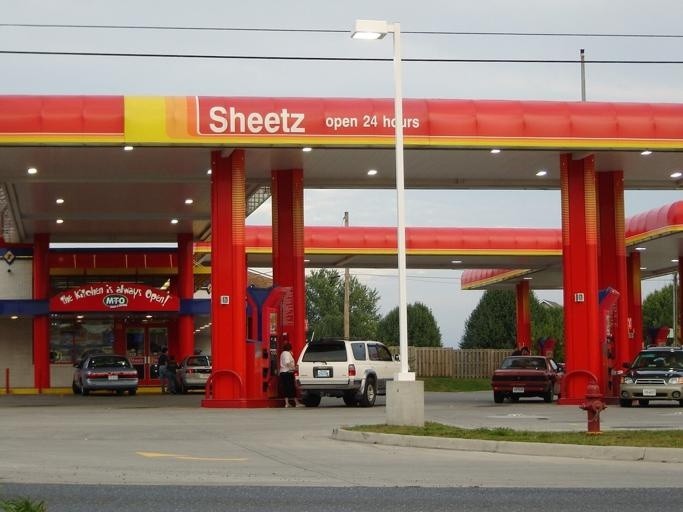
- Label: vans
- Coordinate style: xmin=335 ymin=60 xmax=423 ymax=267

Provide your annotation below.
xmin=294 ymin=339 xmax=410 ymax=407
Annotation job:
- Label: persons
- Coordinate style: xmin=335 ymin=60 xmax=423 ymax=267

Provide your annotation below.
xmin=280 ymin=344 xmax=305 ymax=408
xmin=159 ymin=348 xmax=179 ymax=395
xmin=511 ymin=347 xmax=529 ymax=356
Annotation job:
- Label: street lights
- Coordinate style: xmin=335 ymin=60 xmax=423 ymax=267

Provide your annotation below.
xmin=350 ymin=18 xmax=416 ymax=381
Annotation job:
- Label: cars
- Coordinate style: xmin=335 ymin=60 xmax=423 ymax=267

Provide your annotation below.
xmin=491 ymin=355 xmax=565 ymax=403
xmin=73 ymin=350 xmax=138 ymax=396
xmin=175 ymin=355 xmax=212 ymax=393
xmin=619 ymin=345 xmax=682 ymax=406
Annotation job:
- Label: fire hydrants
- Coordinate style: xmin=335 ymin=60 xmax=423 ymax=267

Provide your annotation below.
xmin=579 ymin=384 xmax=608 ymax=433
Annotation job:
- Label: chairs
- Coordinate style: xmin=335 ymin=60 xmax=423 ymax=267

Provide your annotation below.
xmin=511 ymin=360 xmax=519 ymax=368
